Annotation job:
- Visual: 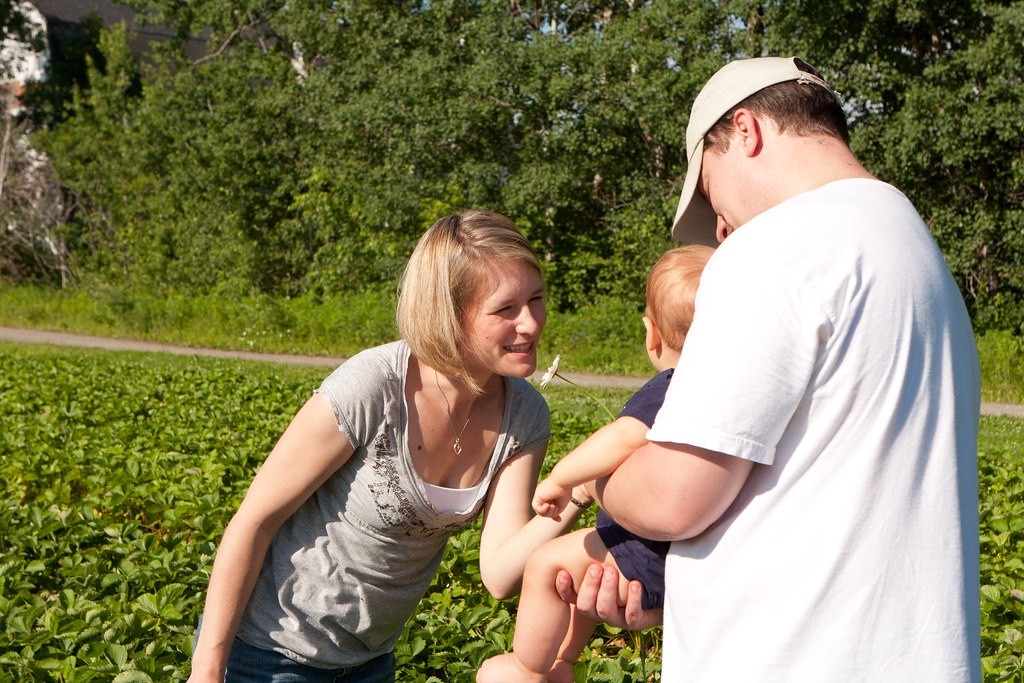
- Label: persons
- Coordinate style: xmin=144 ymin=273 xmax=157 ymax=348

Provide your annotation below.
xmin=576 ymin=56 xmax=983 ymax=683
xmin=187 ymin=209 xmax=594 ymax=683
xmin=475 ymin=245 xmax=718 ymax=683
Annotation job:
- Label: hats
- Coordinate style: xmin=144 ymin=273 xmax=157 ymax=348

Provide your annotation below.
xmin=671 ymin=56 xmax=844 ymax=245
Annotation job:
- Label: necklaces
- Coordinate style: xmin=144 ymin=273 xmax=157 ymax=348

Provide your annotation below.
xmin=435 ymin=370 xmax=477 ymax=455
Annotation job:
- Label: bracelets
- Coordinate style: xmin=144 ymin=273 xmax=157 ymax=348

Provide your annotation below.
xmin=571 ymin=498 xmax=591 ymax=509
